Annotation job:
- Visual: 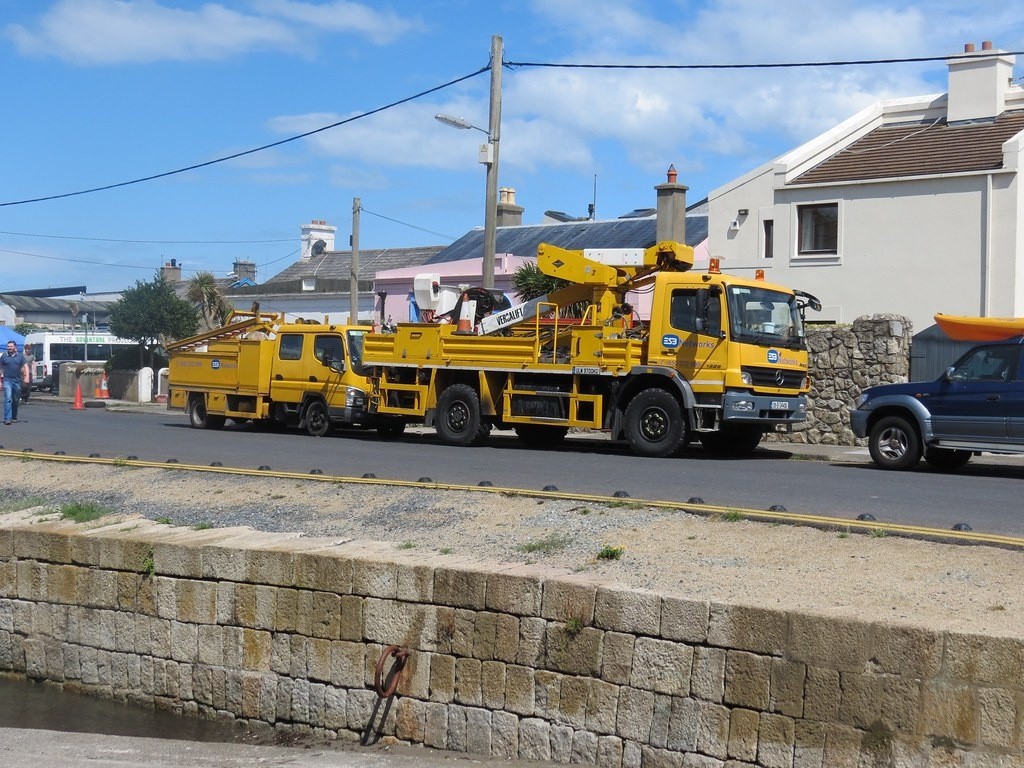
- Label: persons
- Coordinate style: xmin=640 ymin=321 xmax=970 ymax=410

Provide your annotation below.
xmin=18 ymin=344 xmax=36 ymax=406
xmin=0 ymin=340 xmax=30 ymax=425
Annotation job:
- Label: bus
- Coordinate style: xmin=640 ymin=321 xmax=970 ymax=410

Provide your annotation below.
xmin=23 ymin=331 xmax=161 ymax=392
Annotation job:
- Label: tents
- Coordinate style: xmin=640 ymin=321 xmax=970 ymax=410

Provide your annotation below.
xmin=0 ymin=324 xmax=26 ymax=353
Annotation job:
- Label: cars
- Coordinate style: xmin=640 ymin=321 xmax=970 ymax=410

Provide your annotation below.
xmin=847 ymin=333 xmax=1024 ymax=469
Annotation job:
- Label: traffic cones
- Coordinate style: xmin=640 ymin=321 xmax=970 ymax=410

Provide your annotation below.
xmin=94 ymin=372 xmax=111 ymax=398
xmin=69 ymin=384 xmax=85 ymax=410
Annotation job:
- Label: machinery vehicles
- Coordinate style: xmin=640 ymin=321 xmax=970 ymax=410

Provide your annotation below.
xmin=166 ymin=241 xmax=821 ymax=457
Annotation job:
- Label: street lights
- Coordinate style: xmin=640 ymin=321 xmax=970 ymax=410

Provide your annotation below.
xmin=434 ymin=36 xmax=505 ymax=290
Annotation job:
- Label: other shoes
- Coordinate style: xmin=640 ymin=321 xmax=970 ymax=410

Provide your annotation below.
xmin=5 ymin=420 xmax=11 ymax=425
xmin=11 ymin=419 xmax=17 ymax=423
